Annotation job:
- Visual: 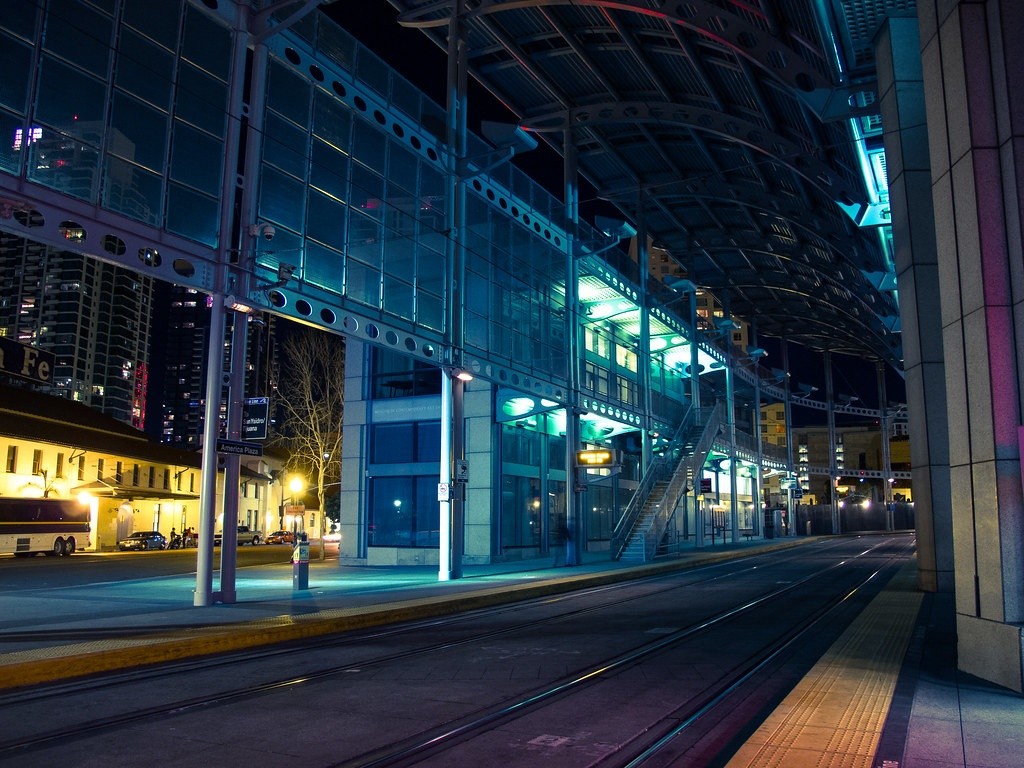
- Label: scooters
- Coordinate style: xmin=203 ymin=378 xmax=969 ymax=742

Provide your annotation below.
xmin=167 ymin=535 xmax=181 ymax=550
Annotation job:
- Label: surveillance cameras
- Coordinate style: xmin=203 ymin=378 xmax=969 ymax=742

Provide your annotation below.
xmin=581 ymin=407 xmax=588 ymax=415
xmin=264 ymin=227 xmax=275 ymax=241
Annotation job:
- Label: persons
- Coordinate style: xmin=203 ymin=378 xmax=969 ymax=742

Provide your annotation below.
xmin=182 ymin=529 xmax=188 ymax=549
xmin=301 ymin=531 xmax=307 ymax=541
xmin=170 ymin=527 xmax=175 ymax=539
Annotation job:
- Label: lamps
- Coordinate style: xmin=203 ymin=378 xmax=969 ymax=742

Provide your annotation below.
xmin=887 ymin=402 xmax=909 ymax=417
xmin=701 ymin=316 xmax=742 ymax=341
xmin=762 ymin=365 xmax=791 ymax=389
xmin=573 ymin=214 xmax=638 ymax=262
xmin=456 ymin=120 xmax=539 ymax=179
xmin=730 ymin=345 xmax=768 ymax=368
xmin=443 ymin=365 xmax=473 ymax=382
xmin=830 ymin=391 xmax=859 ymax=411
xmin=789 ymin=382 xmax=819 ymax=401
xmin=658 ymin=276 xmax=696 ymax=307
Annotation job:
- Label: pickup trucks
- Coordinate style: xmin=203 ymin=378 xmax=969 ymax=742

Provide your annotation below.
xmin=214 ymin=526 xmax=264 ymax=546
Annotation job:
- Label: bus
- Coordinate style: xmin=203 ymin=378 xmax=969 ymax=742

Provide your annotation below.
xmin=0 ymin=497 xmax=92 ymax=558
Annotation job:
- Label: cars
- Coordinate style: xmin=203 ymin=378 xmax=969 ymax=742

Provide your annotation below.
xmin=264 ymin=531 xmax=294 ymax=544
xmin=118 ymin=531 xmax=168 ymax=551
